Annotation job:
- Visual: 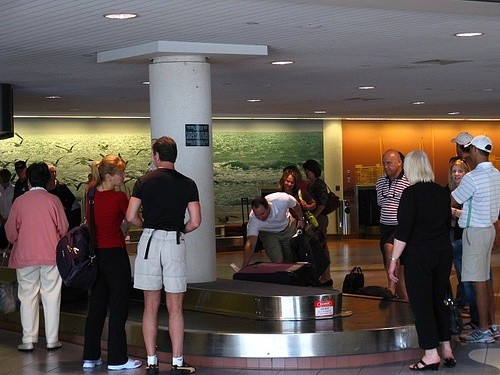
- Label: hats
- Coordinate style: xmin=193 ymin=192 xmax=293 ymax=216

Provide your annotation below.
xmin=451 ymin=132 xmax=473 ymax=145
xmin=464 ymin=135 xmax=493 ymax=152
xmin=302 ymin=159 xmax=321 ymax=177
xmin=14 ymin=161 xmax=26 ymax=170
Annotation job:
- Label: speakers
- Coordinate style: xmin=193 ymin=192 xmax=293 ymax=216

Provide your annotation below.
xmin=0 ymin=83 xmax=14 ymax=140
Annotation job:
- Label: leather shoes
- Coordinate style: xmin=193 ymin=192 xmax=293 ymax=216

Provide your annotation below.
xmin=47 ymin=342 xmax=63 ymax=349
xmin=17 ymin=343 xmax=33 ymax=351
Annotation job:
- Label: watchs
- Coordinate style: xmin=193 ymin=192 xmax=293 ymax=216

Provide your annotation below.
xmin=297 ymin=217 xmax=305 ymax=221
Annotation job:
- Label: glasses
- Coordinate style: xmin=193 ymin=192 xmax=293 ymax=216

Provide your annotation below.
xmin=451 ymin=157 xmax=469 ymax=165
xmin=381 ymin=180 xmax=397 ymax=198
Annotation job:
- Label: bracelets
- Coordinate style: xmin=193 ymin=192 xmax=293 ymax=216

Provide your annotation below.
xmin=389 ymin=257 xmax=398 ymax=262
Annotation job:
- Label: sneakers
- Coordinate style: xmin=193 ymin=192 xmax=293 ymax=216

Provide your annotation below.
xmin=316 ymin=279 xmax=333 ymax=288
xmin=463 ymin=322 xmax=477 ymax=330
xmin=146 ymin=357 xmax=160 ymax=374
xmin=83 ymin=358 xmax=103 ymax=368
xmin=459 ymin=329 xmax=496 ymax=344
xmin=108 ymin=357 xmax=142 ymax=370
xmin=489 ymin=326 xmax=500 ymax=338
xmin=171 ymin=361 xmax=197 ymax=374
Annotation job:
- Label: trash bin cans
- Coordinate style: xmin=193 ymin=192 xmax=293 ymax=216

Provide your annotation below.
xmin=337 ymin=200 xmax=351 ymax=234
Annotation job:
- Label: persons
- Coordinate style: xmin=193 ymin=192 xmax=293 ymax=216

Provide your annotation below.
xmin=47 ymin=166 xmax=77 ymax=211
xmin=282 ymin=165 xmax=316 ymax=226
xmin=388 ymin=150 xmax=456 ymax=371
xmin=451 ymin=132 xmax=477 ymax=318
xmin=82 ymin=156 xmax=143 ymax=371
xmin=444 ymin=155 xmax=473 ymax=319
xmin=0 ymin=170 xmax=15 ymax=248
xmin=303 ymin=160 xmax=333 ymax=288
xmin=273 ymin=170 xmax=304 ymax=263
xmin=376 ymin=148 xmax=412 ymax=301
xmin=451 ymin=134 xmax=500 ymax=343
xmin=126 ymin=137 xmax=201 ymax=375
xmin=241 ymin=192 xmax=305 ymax=264
xmin=5 ymin=162 xmax=70 ymax=352
xmin=12 ymin=161 xmax=29 ymax=203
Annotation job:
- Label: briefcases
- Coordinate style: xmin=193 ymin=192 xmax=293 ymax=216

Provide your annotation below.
xmin=361 ymin=286 xmax=396 ymax=299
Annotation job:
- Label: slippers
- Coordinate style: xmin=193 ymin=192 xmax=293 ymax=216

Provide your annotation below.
xmin=409 ymin=359 xmax=440 ymax=369
xmin=442 ymin=357 xmax=456 ymax=367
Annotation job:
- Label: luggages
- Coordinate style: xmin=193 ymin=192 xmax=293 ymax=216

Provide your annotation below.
xmin=241 ymin=197 xmax=263 ymax=253
xmin=233 ymin=261 xmax=305 ymax=286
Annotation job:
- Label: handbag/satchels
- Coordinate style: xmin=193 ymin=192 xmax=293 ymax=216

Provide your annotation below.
xmin=315 ymin=179 xmax=340 ymax=215
xmin=293 ymin=224 xmax=331 ymax=280
xmin=446 ymin=299 xmax=463 ymax=335
xmin=56 ymin=225 xmax=101 ymax=288
xmin=342 ymin=267 xmax=364 ymax=293
xmin=298 ymin=189 xmax=320 ymax=229
